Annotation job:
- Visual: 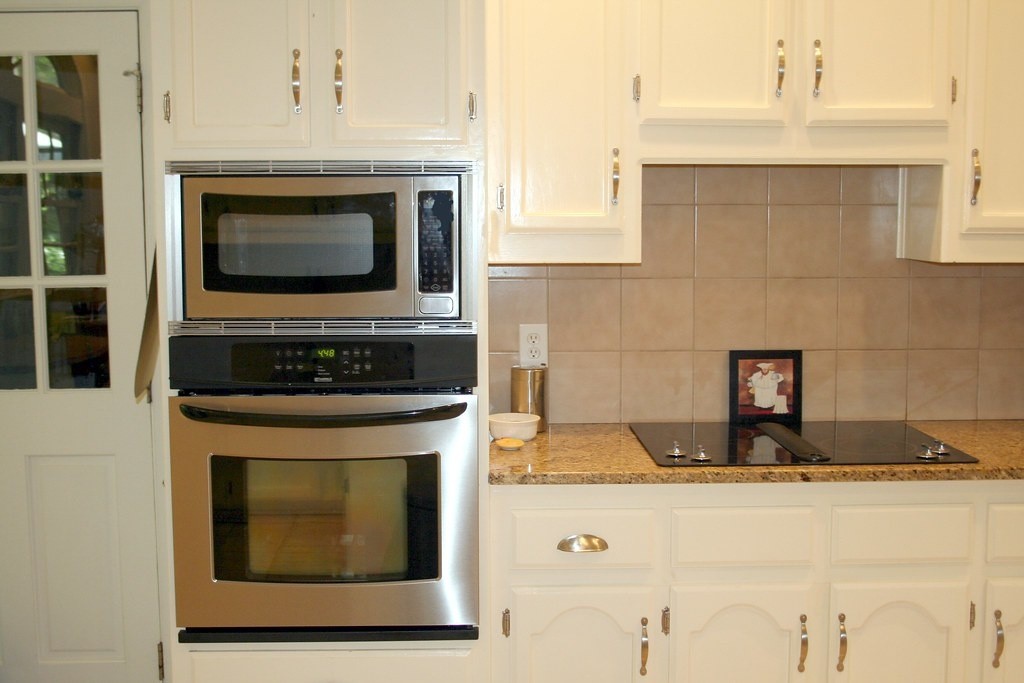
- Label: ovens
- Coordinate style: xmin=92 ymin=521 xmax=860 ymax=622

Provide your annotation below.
xmin=167 ymin=333 xmax=481 ymax=644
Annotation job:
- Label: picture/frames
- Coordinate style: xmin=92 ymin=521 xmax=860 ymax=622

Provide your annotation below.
xmin=729 ymin=349 xmax=803 ymax=428
xmin=727 ymin=428 xmax=802 ymax=466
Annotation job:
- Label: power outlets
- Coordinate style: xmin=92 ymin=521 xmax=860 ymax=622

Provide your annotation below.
xmin=518 ymin=323 xmax=549 ymax=369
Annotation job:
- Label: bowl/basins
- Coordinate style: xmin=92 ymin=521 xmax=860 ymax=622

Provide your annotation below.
xmin=488 ymin=412 xmax=541 ymax=442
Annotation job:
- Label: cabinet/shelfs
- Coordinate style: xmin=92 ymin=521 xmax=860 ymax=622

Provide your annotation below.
xmin=148 ymin=0 xmax=478 ymax=159
xmin=640 ymin=1 xmax=951 ymax=166
xmin=833 ymin=479 xmax=985 ymax=683
xmin=492 ymin=478 xmax=670 ymax=683
xmin=984 ymin=479 xmax=1024 ymax=683
xmin=902 ymin=1 xmax=1024 ymax=264
xmin=487 ymin=0 xmax=641 ymax=265
xmin=668 ymin=481 xmax=833 ymax=683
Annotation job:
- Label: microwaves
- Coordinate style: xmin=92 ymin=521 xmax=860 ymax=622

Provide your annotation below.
xmin=181 ymin=174 xmax=462 ymax=321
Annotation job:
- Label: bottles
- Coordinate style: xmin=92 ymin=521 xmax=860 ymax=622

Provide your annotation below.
xmin=509 ymin=366 xmax=547 ymax=433
